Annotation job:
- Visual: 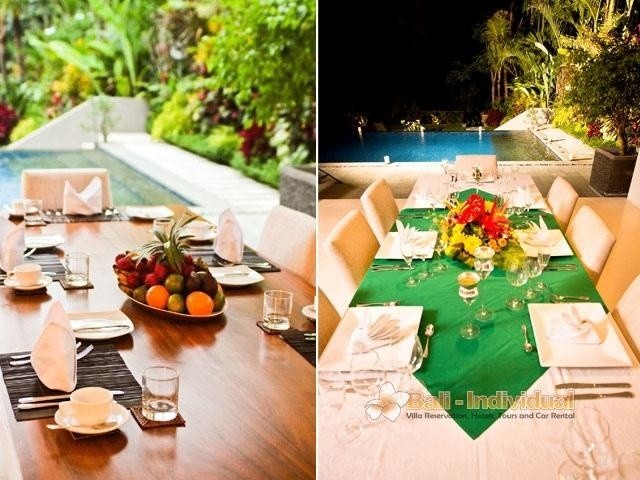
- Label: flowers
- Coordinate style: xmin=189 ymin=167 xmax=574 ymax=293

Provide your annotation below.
xmin=566 ymin=3 xmax=640 ymax=155
xmin=428 ymin=190 xmax=526 ymax=270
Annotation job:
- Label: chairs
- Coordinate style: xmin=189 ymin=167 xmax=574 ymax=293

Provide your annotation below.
xmin=452 ymin=154 xmax=499 ymax=178
xmin=317 ymin=286 xmax=342 ymax=357
xmin=546 ymin=177 xmax=578 ymax=233
xmin=562 ymin=204 xmax=616 ymax=290
xmin=611 ymin=270 xmax=640 ymax=358
xmin=255 ymin=206 xmax=313 ymax=286
xmin=17 ymin=167 xmax=115 ymax=210
xmin=326 ymin=208 xmax=380 ymax=287
xmin=355 ymin=179 xmax=399 ymax=239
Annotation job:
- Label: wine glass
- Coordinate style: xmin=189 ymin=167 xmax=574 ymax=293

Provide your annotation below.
xmin=401 ymin=156 xmax=551 ymax=339
xmin=560 ymin=406 xmax=609 ymax=480
xmin=319 ymin=329 xmax=422 ymax=439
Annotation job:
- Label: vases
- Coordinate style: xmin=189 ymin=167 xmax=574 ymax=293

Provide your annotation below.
xmin=586 ymin=148 xmax=638 ymax=198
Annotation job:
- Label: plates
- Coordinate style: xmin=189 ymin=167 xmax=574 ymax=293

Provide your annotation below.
xmin=122 ymin=290 xmax=229 ymax=321
xmin=375 ymin=228 xmax=440 ymax=262
xmin=53 ymin=403 xmax=128 ymax=433
xmin=68 ymin=310 xmax=135 ymax=342
xmin=526 ymin=303 xmax=632 ymax=369
xmin=320 ymin=303 xmax=423 ymax=372
xmin=513 ymin=227 xmax=573 ymax=257
xmin=25 ymin=232 xmax=64 ymax=249
xmin=208 ymin=264 xmax=264 ymax=286
xmin=181 ymin=228 xmax=216 ymax=243
xmin=124 ymin=204 xmax=176 ymax=220
xmin=5 ymin=274 xmax=51 ymax=291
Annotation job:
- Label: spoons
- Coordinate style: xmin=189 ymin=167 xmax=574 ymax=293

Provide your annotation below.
xmin=548 ymin=293 xmax=591 ymax=303
xmin=520 ymin=323 xmax=533 ymax=352
xmin=422 ymin=322 xmax=434 ymax=358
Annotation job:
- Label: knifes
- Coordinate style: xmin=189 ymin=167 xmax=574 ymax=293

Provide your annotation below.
xmin=564 ymin=392 xmax=633 ymax=401
xmin=371 ymin=264 xmax=414 ymax=271
xmin=15 ymin=388 xmax=126 ymax=410
xmin=556 ymin=381 xmax=630 ymax=390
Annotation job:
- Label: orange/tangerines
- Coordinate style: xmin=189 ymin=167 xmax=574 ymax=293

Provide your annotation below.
xmin=113 ymin=250 xmax=226 ymax=316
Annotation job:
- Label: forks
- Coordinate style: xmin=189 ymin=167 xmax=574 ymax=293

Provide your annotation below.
xmin=9 ymin=339 xmax=94 ymax=366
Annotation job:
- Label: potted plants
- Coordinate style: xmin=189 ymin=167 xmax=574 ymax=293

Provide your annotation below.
xmin=202 ymin=1 xmax=316 ymax=213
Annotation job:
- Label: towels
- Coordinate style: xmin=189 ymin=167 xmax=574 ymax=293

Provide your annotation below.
xmin=60 ymin=177 xmax=104 ymax=217
xmin=542 ymin=307 xmax=613 ymax=347
xmin=517 ymin=186 xmax=542 ymax=210
xmin=1 ymin=218 xmax=26 ymax=271
xmin=208 ymin=209 xmax=245 ymax=264
xmin=349 ymin=308 xmax=406 ymax=355
xmin=395 ymin=217 xmax=430 ymax=256
xmin=522 ymin=217 xmax=560 ymax=248
xmin=28 ymin=302 xmax=79 ymax=394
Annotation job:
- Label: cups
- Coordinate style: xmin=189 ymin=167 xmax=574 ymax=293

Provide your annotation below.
xmin=64 ymin=251 xmax=90 ymax=286
xmin=187 ymin=222 xmax=213 ymax=237
xmin=8 ymin=263 xmax=42 ymax=284
xmin=153 ymin=218 xmax=175 ymax=244
xmin=25 ymin=200 xmax=44 ymax=224
xmin=384 ymin=156 xmax=390 ymax=164
xmin=262 ymin=289 xmax=293 ymax=331
xmin=60 ymin=385 xmax=112 ymax=421
xmin=139 ymin=365 xmax=180 ymax=422
